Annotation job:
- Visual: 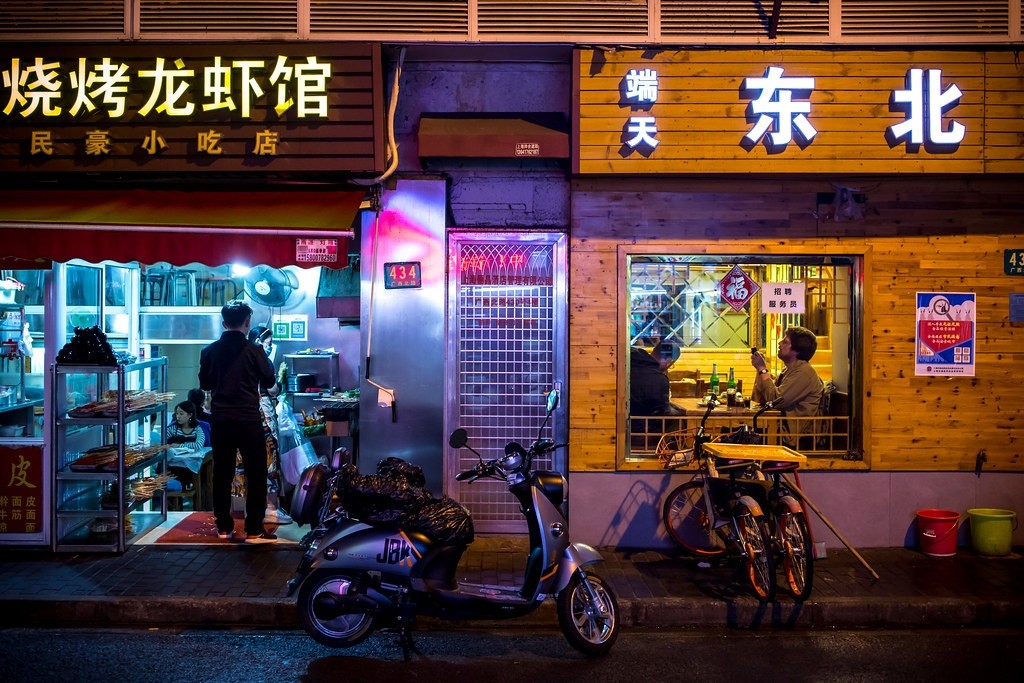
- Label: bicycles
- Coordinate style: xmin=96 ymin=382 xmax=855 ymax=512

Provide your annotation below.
xmin=655 ymin=393 xmax=815 ymax=603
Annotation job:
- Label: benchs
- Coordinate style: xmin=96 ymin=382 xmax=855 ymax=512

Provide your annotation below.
xmin=152 ymin=479 xmax=197 ymax=511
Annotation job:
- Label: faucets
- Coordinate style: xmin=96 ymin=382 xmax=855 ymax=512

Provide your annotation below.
xmin=977 ymin=448 xmax=988 ymax=463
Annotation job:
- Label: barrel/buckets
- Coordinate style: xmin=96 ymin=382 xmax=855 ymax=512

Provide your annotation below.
xmin=916 ymin=509 xmax=961 ymax=555
xmin=967 ymin=508 xmax=1018 ymax=556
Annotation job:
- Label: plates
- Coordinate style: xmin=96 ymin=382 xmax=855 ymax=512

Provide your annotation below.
xmin=697 ymin=401 xmax=720 ymax=406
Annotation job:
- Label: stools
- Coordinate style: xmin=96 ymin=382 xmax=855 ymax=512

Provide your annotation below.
xmin=166 ymin=266 xmax=199 ymax=305
xmin=201 ymin=276 xmax=237 ymax=306
xmin=142 ymin=272 xmax=165 ymax=308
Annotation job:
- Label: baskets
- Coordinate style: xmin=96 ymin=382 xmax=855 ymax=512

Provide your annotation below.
xmin=655 ymin=425 xmax=702 ymax=469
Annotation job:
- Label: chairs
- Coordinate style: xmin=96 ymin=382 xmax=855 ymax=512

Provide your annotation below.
xmin=702 ymin=376 xmax=745 ymax=399
xmin=665 ymin=367 xmax=701 ymax=380
xmin=669 ymin=376 xmax=702 ymax=398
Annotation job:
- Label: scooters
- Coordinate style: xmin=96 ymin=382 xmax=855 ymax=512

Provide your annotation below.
xmin=286 ymin=387 xmax=622 ymax=663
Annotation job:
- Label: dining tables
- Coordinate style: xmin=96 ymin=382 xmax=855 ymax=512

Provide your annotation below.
xmin=152 ymin=444 xmax=212 ymax=510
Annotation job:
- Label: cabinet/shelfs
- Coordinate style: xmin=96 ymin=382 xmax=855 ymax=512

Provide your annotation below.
xmin=54 ymin=354 xmax=171 ymax=553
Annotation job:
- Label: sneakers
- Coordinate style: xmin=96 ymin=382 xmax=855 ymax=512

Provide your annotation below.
xmin=263 ymin=508 xmax=293 ymax=525
xmin=217 ymin=528 xmax=232 ymax=538
xmin=245 ymin=531 xmax=278 ymax=544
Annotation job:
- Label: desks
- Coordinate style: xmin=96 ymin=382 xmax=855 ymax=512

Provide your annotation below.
xmin=669 ymin=392 xmax=782 ymax=446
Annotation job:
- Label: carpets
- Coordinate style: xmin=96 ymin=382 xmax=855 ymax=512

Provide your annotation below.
xmin=152 ymin=506 xmax=282 ymax=543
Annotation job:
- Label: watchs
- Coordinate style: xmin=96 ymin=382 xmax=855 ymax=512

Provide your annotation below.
xmin=759 ymin=369 xmax=768 ymax=376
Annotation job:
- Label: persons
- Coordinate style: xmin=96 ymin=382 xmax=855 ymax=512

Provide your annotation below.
xmin=198 ymin=300 xmax=277 ymax=544
xmin=155 ymin=400 xmax=205 ymax=478
xmin=150 ymin=412 xmax=182 ymax=511
xmin=631 ymin=285 xmax=685 ymax=346
xmin=751 ymin=326 xmax=823 ymax=450
xmin=171 ymin=388 xmax=211 ymax=445
xmin=232 ymin=326 xmax=288 ymax=496
xmin=631 ymin=339 xmax=681 ymax=446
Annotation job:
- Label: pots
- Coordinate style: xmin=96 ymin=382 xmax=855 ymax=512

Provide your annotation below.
xmin=285 ymin=373 xmax=317 ymax=392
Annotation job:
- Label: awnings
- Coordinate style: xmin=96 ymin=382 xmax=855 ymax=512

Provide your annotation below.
xmin=0 ymin=188 xmax=368 ymax=269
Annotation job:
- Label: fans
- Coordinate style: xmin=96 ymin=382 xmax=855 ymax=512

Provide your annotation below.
xmin=235 ymin=263 xmax=296 ymax=310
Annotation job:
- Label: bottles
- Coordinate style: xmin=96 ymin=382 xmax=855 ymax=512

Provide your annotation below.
xmin=727 ymin=368 xmax=737 ymax=393
xmin=710 ymin=364 xmax=720 ymax=396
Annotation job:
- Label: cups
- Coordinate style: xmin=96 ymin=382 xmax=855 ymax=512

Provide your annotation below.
xmin=727 ymin=388 xmax=736 ymax=406
xmin=745 ymin=396 xmax=750 ymax=407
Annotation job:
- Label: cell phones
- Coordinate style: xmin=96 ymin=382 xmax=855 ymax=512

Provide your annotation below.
xmin=751 ymin=347 xmax=757 ymax=354
